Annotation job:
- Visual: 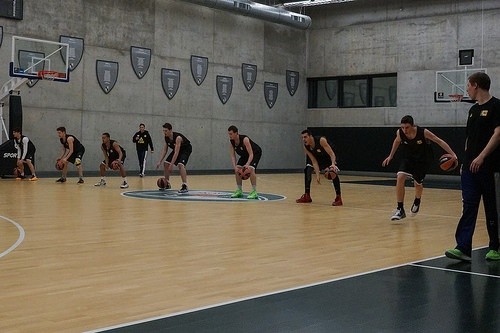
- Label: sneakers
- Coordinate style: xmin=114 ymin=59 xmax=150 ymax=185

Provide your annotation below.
xmin=445 ymin=249 xmax=472 ymax=262
xmin=332 ymin=197 xmax=343 ymax=206
xmin=391 ymin=207 xmax=406 ymax=221
xmin=296 ymin=194 xmax=312 ymax=203
xmin=410 ymin=202 xmax=420 ymax=217
xmin=485 ymin=250 xmax=500 ymax=260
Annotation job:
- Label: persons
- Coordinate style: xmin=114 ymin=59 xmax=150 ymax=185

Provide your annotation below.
xmin=295 ymin=130 xmax=343 ymax=206
xmin=56 ymin=127 xmax=86 ymax=183
xmin=156 ymin=123 xmax=192 ymax=193
xmin=94 ymin=133 xmax=129 ymax=188
xmin=133 ymin=124 xmax=154 ymax=177
xmin=445 ymin=72 xmax=500 ymax=261
xmin=227 ymin=126 xmax=262 ymax=199
xmin=12 ymin=128 xmax=38 ymax=181
xmin=382 ymin=115 xmax=458 ymax=221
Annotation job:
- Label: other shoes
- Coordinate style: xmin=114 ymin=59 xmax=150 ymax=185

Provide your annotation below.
xmin=120 ymin=181 xmax=129 ymax=189
xmin=177 ymin=184 xmax=189 ymax=193
xmin=230 ymin=188 xmax=244 ymax=198
xmin=139 ymin=173 xmax=144 ymax=177
xmin=246 ymin=189 xmax=258 ymax=199
xmin=28 ymin=175 xmax=37 ymax=181
xmin=77 ymin=178 xmax=84 ymax=184
xmin=16 ymin=175 xmax=25 ymax=180
xmin=159 ymin=183 xmax=171 ymax=191
xmin=93 ymin=179 xmax=106 ymax=186
xmin=55 ymin=177 xmax=66 ymax=183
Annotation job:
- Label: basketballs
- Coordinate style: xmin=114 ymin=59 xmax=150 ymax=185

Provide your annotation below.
xmin=238 ymin=166 xmax=250 ymax=180
xmin=111 ymin=160 xmax=121 ymax=170
xmin=440 ymin=154 xmax=457 ymax=171
xmin=14 ymin=167 xmax=22 ymax=176
xmin=157 ymin=177 xmax=167 ymax=188
xmin=324 ymin=166 xmax=337 ymax=180
xmin=55 ymin=160 xmax=65 ymax=170
